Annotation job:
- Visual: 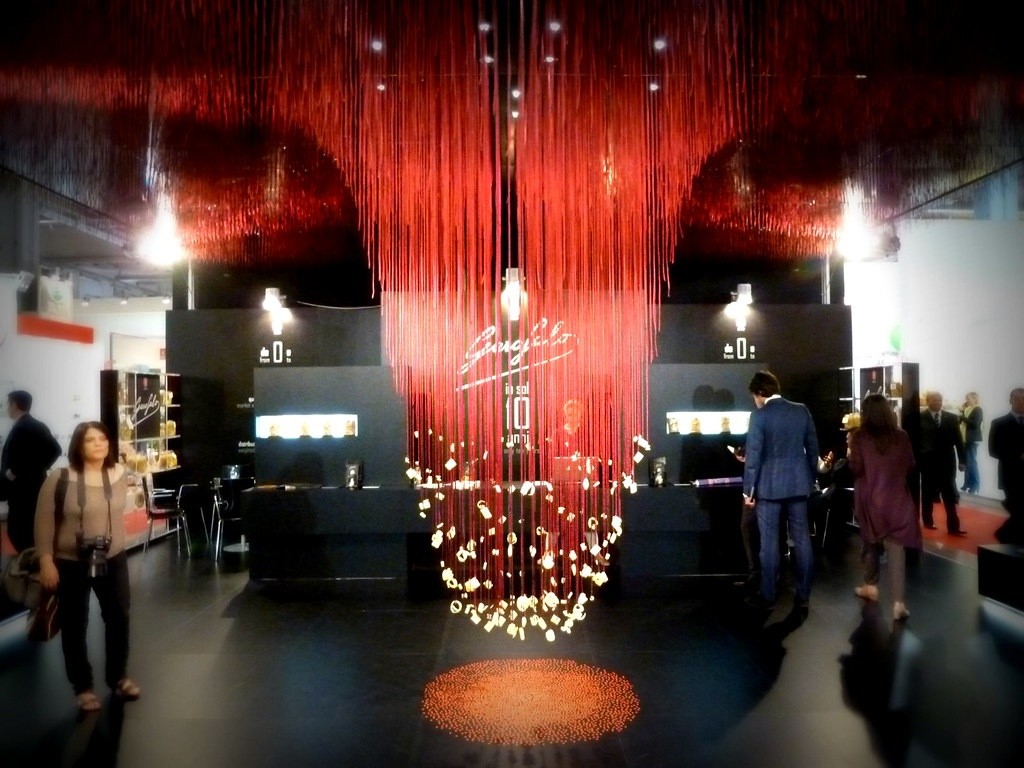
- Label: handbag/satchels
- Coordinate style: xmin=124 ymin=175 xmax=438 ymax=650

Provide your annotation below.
xmin=27 ymin=593 xmax=60 ymax=641
xmin=0 ymin=547 xmax=40 ymax=610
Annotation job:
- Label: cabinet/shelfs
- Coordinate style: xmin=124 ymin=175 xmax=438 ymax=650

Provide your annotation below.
xmin=859 ymin=362 xmax=921 ymax=434
xmin=101 ymin=371 xmax=183 ymax=473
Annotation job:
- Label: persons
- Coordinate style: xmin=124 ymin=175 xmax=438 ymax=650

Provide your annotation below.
xmin=0 ymin=390 xmax=61 ymax=617
xmin=728 ymin=444 xmax=833 ymax=591
xmin=848 ymin=395 xmax=921 ymax=619
xmin=989 ymin=389 xmax=1024 ymax=547
xmin=556 ymin=401 xmax=586 ymax=456
xmin=846 ymin=427 xmax=885 ymax=560
xmin=742 ymin=373 xmax=819 ymax=608
xmin=864 ymin=370 xmax=882 ymax=399
xmin=35 ymin=422 xmax=140 ymax=710
xmin=917 ymin=391 xmax=967 ymax=534
xmin=958 ymin=392 xmax=983 ymax=492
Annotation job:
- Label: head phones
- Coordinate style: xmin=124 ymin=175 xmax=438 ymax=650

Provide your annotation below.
xmin=82 ymin=536 xmax=112 ymax=578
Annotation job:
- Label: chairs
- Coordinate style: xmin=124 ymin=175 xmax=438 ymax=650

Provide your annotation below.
xmin=821 ymin=487 xmax=861 ymax=551
xmin=144 ymin=464 xmax=248 ymax=563
xmin=783 ymin=520 xmax=817 ymax=559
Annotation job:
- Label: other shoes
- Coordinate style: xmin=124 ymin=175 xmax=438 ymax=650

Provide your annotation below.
xmin=923 ymin=522 xmax=937 ymax=529
xmin=948 ymin=529 xmax=967 ymax=534
xmin=893 ymin=606 xmax=910 ymax=620
xmin=854 ymin=584 xmax=879 ymax=602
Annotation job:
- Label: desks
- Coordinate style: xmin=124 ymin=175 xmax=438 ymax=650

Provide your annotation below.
xmin=241 ymin=483 xmax=760 ymax=600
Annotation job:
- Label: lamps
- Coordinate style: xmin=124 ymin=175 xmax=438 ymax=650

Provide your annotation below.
xmin=727 ymin=284 xmax=757 ymax=306
xmin=262 ymin=289 xmax=291 ymax=312
xmin=502 ymin=267 xmax=526 ymax=321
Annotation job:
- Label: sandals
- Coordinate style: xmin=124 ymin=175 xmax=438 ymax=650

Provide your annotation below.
xmin=77 ymin=689 xmax=102 ymax=711
xmin=107 ymin=676 xmax=140 ymax=697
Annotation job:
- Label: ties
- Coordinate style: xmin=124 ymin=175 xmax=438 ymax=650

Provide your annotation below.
xmin=934 ymin=414 xmax=939 ymax=427
